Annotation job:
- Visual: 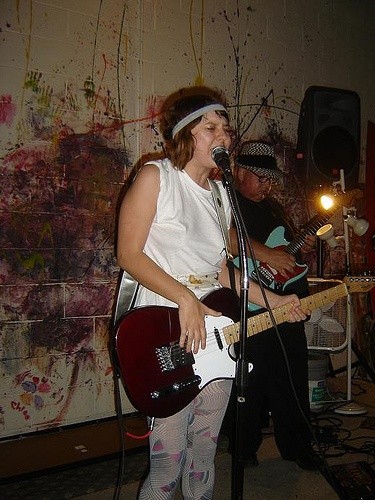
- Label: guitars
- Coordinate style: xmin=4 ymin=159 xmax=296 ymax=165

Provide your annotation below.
xmin=230 ymin=187 xmax=363 ymax=312
xmin=108 ymin=272 xmax=375 ymax=421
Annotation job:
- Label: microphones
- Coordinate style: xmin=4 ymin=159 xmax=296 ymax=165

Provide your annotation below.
xmin=212 ymin=145 xmax=235 ymax=183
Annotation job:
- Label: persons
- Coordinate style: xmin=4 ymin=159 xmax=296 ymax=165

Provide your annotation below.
xmin=227 ymin=141 xmax=321 ymax=470
xmin=116 ymin=87 xmax=312 ymax=500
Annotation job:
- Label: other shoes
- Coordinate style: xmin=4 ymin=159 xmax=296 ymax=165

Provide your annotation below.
xmin=296 ymin=452 xmax=323 ymax=470
xmin=244 ymin=456 xmax=259 ymax=468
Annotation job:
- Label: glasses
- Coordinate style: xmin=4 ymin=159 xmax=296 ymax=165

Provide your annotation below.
xmin=249 ymin=170 xmax=279 ymax=185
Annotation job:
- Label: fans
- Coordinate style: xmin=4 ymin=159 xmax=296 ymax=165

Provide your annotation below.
xmin=304 ymin=278 xmax=348 ymax=350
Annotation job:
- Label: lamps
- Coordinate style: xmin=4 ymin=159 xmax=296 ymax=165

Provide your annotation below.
xmin=316 ymin=205 xmax=370 ymax=415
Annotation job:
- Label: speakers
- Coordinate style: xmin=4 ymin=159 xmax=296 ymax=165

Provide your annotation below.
xmin=296 ymin=85 xmax=362 ymax=192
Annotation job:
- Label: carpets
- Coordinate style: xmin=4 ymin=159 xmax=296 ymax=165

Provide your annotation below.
xmin=0 ymin=433 xmax=375 ymax=500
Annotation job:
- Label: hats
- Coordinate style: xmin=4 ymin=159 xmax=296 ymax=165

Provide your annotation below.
xmin=234 ymin=142 xmax=284 ymax=176
